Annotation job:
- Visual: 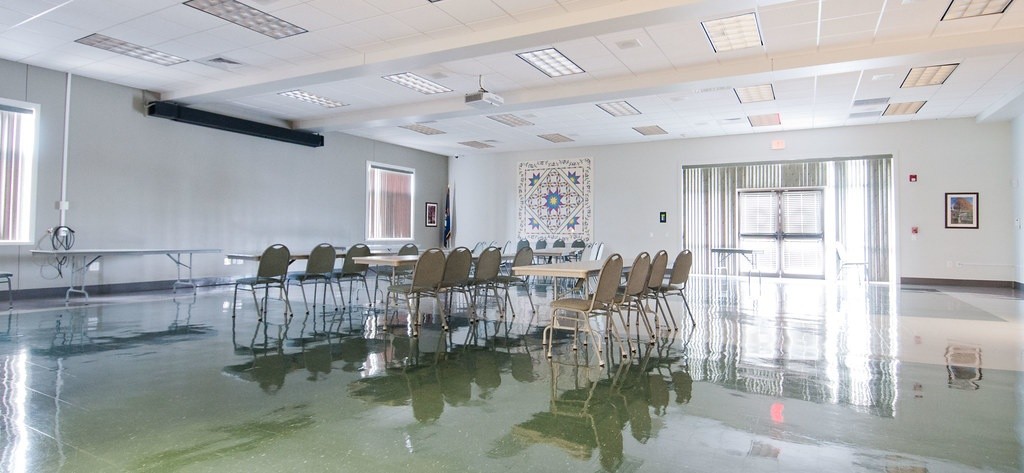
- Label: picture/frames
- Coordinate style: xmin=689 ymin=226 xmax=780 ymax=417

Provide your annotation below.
xmin=424 ymin=203 xmax=439 ymax=225
xmin=945 ymin=193 xmax=980 ymax=228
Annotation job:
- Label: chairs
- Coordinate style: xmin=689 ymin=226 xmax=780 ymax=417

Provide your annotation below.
xmin=232 ymin=239 xmax=699 ymax=364
xmin=836 ymin=240 xmax=870 ymax=282
xmin=0 ymin=271 xmax=14 ymax=309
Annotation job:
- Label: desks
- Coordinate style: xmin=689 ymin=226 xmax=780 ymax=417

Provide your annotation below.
xmin=367 ymin=247 xmax=467 ymax=300
xmin=350 ymin=247 xmax=497 ymax=340
xmin=511 ymin=258 xmax=658 ymax=359
xmin=532 ymin=247 xmax=584 ymax=291
xmin=28 ymin=246 xmax=220 ymax=310
xmin=711 ymin=247 xmax=765 ymax=278
xmin=224 ymin=248 xmax=348 ymax=352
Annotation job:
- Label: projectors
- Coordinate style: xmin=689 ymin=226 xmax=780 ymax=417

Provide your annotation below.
xmin=464 ymin=93 xmax=504 ymax=110
